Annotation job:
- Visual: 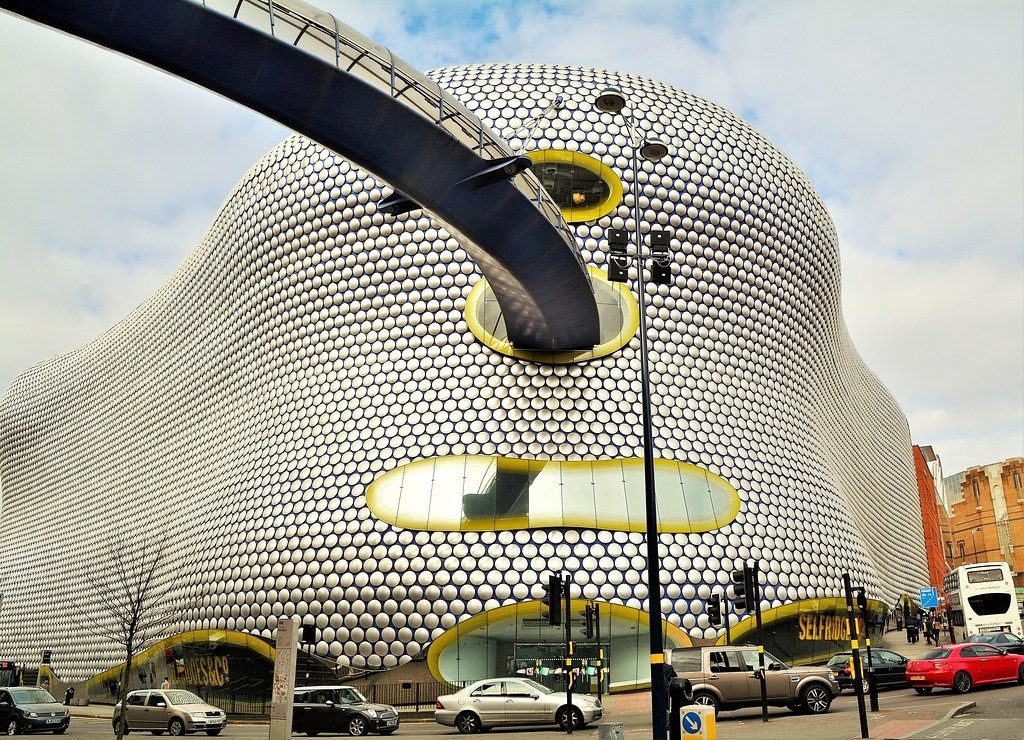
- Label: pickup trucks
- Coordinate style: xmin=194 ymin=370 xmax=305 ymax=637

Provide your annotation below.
xmin=673 ymin=645 xmax=841 ymax=715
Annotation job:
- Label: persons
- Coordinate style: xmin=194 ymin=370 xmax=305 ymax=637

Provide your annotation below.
xmin=663 ymin=651 xmax=678 ymax=731
xmin=896 ymin=603 xmax=945 ymax=647
xmin=41 ymin=680 xmax=50 ymax=690
xmin=109 ymin=676 xmax=118 ymax=697
xmin=318 ymin=693 xmax=330 ymax=703
xmin=161 ymin=677 xmax=171 ymax=689
xmin=137 ymin=662 xmax=156 ymax=685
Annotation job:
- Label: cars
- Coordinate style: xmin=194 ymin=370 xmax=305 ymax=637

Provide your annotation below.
xmin=292 ymin=686 xmax=400 ymax=736
xmin=904 ymin=643 xmax=1024 ymax=695
xmin=110 ymin=689 xmax=228 ymax=736
xmin=434 ymin=678 xmax=603 ymax=733
xmin=962 ymin=631 xmax=1024 ymax=656
xmin=828 ymin=648 xmax=911 ymax=694
xmin=0 ymin=686 xmax=71 ymax=736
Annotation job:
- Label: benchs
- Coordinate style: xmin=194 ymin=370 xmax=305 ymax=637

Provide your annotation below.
xmin=130 ymin=696 xmax=144 ymax=705
xmin=294 ymin=692 xmax=309 ymax=703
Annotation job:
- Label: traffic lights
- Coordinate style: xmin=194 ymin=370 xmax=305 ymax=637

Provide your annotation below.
xmin=708 ymin=593 xmax=722 ymax=625
xmin=579 ymin=604 xmax=593 ymax=639
xmin=732 ymin=563 xmax=754 ymax=614
xmin=541 ymin=575 xmax=562 ymax=626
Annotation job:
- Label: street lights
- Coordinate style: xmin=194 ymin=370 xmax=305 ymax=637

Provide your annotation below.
xmin=593 ymin=85 xmax=669 ymax=739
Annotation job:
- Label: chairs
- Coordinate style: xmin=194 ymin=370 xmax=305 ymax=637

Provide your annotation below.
xmin=308 ymin=692 xmax=317 ymax=703
xmin=978 ymin=637 xmax=986 ymax=642
xmin=318 ymin=692 xmax=326 ymax=703
xmin=969 ymin=650 xmax=976 ymax=656
xmin=149 ymin=695 xmax=157 ymax=705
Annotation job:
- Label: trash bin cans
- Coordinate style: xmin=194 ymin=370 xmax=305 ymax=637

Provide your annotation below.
xmin=668 ymin=677 xmax=693 ymax=740
xmin=62 ymin=687 xmax=75 ymax=705
xmin=896 ymin=617 xmax=902 ymax=631
xmin=597 ymin=722 xmax=624 ymax=740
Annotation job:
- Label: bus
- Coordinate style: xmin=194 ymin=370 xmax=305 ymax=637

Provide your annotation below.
xmin=942 ymin=561 xmax=1024 ymax=644
